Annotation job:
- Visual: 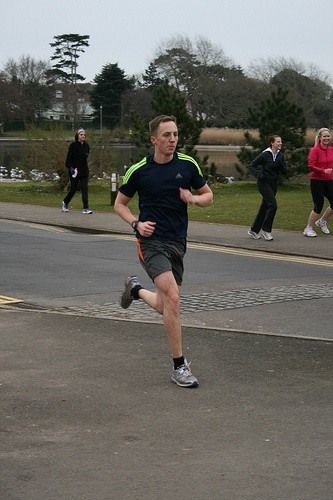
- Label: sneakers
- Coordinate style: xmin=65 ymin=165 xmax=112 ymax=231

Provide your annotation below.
xmin=315 ymin=219 xmax=330 ymax=234
xmin=61 ymin=201 xmax=68 ymax=212
xmin=120 ymin=276 xmax=143 ymax=308
xmin=248 ymin=229 xmax=262 ymax=239
xmin=303 ymin=228 xmax=317 ymax=237
xmin=261 ymin=229 xmax=274 ymax=241
xmin=171 ymin=358 xmax=199 ymax=388
xmin=83 ymin=209 xmax=92 ymax=214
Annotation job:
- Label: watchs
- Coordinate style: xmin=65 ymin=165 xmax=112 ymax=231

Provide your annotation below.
xmin=131 ymin=220 xmax=140 ymax=232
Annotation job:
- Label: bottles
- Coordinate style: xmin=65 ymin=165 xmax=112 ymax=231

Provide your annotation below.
xmin=72 ymin=167 xmax=78 ymax=178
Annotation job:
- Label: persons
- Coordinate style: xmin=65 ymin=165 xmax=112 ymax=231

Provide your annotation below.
xmin=247 ymin=134 xmax=289 ymax=241
xmin=62 ymin=128 xmax=93 ymax=214
xmin=113 ymin=114 xmax=213 ymax=390
xmin=303 ymin=128 xmax=333 ymax=237
